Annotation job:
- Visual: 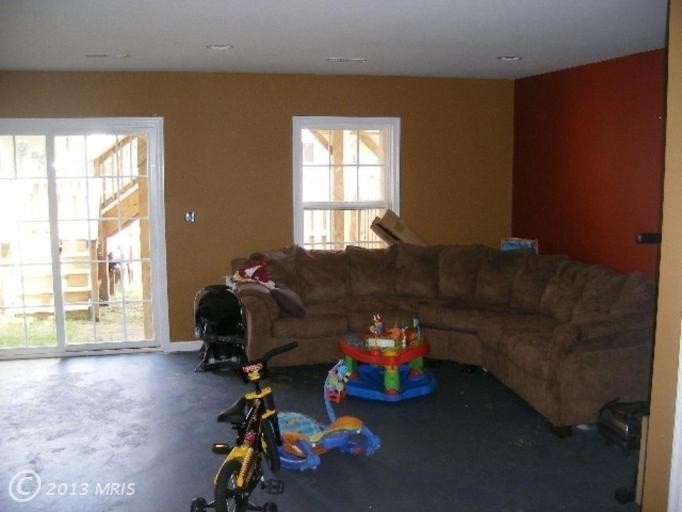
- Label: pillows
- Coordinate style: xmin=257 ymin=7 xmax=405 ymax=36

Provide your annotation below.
xmin=267 ymin=244 xmax=646 ymax=330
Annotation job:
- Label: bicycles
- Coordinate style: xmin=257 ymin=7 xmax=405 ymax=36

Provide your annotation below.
xmin=191 ymin=340 xmax=298 ymax=511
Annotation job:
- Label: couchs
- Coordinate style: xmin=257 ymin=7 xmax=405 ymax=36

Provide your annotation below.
xmin=227 ymin=247 xmax=656 ymax=439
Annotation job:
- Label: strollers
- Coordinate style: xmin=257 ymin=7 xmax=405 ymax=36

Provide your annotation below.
xmin=192 ymin=283 xmax=249 ymax=373
